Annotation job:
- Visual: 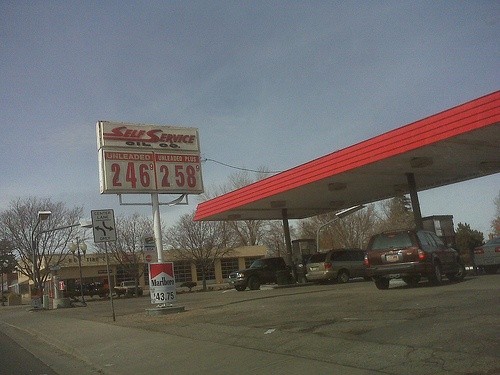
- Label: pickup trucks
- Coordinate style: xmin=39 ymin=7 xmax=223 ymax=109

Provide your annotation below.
xmin=113 ymin=280 xmax=143 ymax=296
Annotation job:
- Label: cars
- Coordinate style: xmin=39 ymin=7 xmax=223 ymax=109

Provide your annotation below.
xmin=472 ymin=238 xmax=500 ymax=273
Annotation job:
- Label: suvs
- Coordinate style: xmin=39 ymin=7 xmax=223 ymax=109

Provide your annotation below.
xmin=228 ymin=257 xmax=297 ymax=292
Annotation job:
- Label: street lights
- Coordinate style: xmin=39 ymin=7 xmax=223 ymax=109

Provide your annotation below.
xmin=0 ymin=261 xmax=9 ymax=306
xmin=32 ymin=209 xmax=52 ymax=285
xmin=68 ymin=236 xmax=87 ymax=304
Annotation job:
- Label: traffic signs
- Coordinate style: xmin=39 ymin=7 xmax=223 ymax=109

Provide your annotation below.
xmin=90 ymin=209 xmax=117 ymax=242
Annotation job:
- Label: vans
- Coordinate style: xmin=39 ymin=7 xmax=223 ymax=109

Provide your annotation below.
xmin=303 ymin=246 xmax=367 ymax=284
xmin=363 ymin=229 xmax=465 ymax=289
